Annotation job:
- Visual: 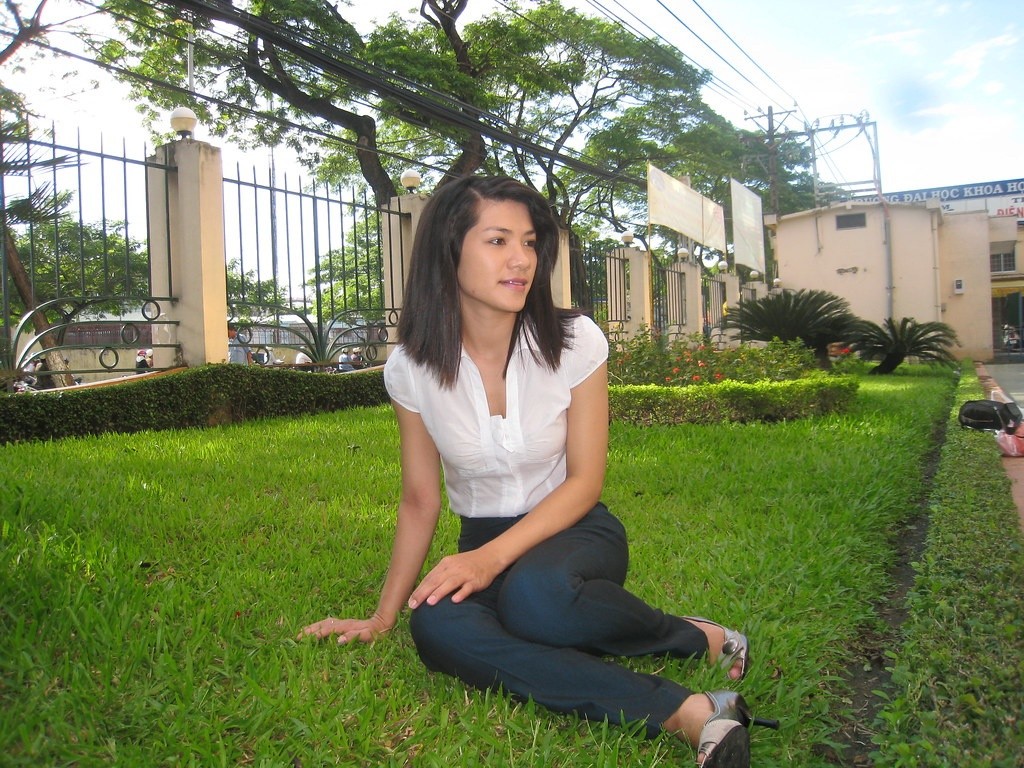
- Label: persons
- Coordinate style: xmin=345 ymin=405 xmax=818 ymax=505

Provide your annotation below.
xmin=264 ymin=347 xmax=275 ymax=365
xmin=135 ymin=349 xmax=153 ymax=374
xmin=298 ymin=176 xmax=781 ymax=768
xmin=228 ymin=325 xmax=253 ymax=364
xmin=296 ymin=346 xmax=314 ymax=363
xmin=338 ymin=347 xmax=363 ymax=371
xmin=20 ymin=353 xmax=70 ymax=390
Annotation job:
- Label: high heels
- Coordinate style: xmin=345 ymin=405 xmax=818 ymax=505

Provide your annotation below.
xmin=697 ymin=690 xmax=780 ymax=768
xmin=681 ymin=616 xmax=748 ymax=681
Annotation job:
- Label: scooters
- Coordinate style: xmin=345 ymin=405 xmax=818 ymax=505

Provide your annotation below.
xmin=1003 ymin=324 xmax=1021 ymax=350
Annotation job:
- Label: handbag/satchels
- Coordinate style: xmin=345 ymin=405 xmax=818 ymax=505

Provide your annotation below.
xmin=958 ymin=400 xmax=1022 ymax=435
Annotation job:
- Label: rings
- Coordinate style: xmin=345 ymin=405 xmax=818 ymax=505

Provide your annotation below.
xmin=331 ymin=620 xmax=334 ymax=624
